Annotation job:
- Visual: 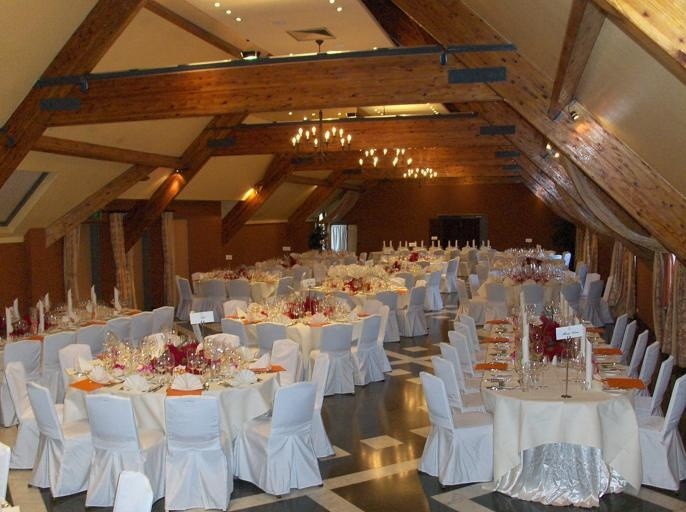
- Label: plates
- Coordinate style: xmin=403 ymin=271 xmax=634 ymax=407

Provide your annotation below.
xmin=85 ymin=375 xmax=111 ymax=384
xmin=587 ymin=334 xmax=629 ymax=396
xmin=487 ymin=320 xmax=517 ymax=390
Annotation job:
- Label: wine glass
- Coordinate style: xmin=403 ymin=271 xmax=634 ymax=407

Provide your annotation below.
xmin=103 ymin=335 xmax=246 ymax=386
xmin=248 ymin=294 xmax=348 ymax=324
xmin=509 ymin=300 xmax=590 ymax=394
xmin=12 ymin=297 xmax=115 ymax=336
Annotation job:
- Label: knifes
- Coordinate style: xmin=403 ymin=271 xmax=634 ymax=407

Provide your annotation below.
xmin=152 ymin=382 xmax=164 ymax=392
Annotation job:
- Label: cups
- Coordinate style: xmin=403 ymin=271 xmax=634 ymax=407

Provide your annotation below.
xmin=503 ymin=262 xmax=566 ymax=283
xmin=324 ymin=275 xmax=389 ymax=294
xmin=507 ymin=247 xmax=545 ymax=257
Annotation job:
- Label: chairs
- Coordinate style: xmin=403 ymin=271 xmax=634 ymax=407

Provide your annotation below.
xmin=0 ymin=233 xmax=686 ymax=511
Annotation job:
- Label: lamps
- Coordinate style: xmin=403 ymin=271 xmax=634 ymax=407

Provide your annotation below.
xmin=399 ymin=166 xmax=440 ymax=181
xmin=285 ymin=38 xmax=352 ymax=165
xmin=358 ymin=102 xmax=414 ymax=173
xmin=566 ymin=102 xmax=582 ymax=122
